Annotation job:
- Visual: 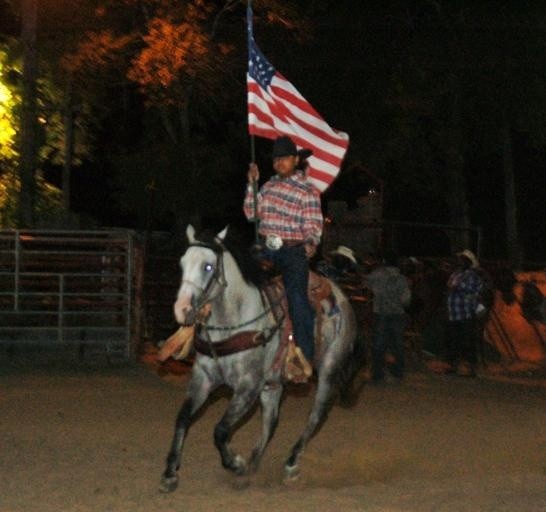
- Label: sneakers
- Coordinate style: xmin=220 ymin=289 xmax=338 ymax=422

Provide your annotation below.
xmin=159 ymin=341 xmax=194 ymax=364
xmin=289 ymin=357 xmax=314 ymax=374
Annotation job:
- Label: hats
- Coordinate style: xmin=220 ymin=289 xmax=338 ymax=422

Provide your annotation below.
xmin=329 ymin=246 xmax=356 ymax=264
xmin=264 ymin=136 xmax=313 ymax=159
xmin=458 ymin=249 xmax=479 ymax=269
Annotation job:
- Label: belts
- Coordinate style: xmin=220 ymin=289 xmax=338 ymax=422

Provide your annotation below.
xmin=258 ymin=233 xmax=304 ymax=250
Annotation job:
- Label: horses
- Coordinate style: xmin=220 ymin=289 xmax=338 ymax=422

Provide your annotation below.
xmin=157 ymin=222 xmax=359 ymax=496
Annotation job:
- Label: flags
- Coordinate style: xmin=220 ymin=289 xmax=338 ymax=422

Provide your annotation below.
xmin=246 ymin=32 xmax=351 ymax=194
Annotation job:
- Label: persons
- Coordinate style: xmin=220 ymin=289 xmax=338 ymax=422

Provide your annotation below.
xmin=312 ymin=243 xmax=496 ymax=409
xmin=239 ymin=135 xmax=326 ymax=383
